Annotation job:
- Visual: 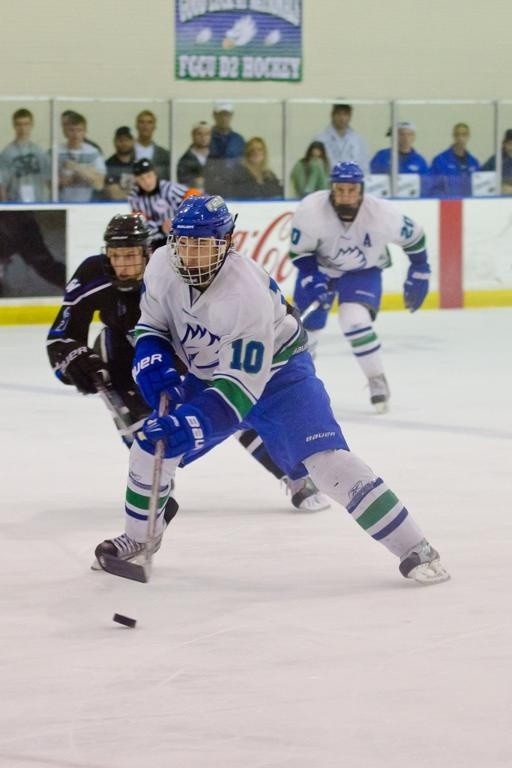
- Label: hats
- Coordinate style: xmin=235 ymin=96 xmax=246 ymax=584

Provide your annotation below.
xmin=213 ymin=98 xmax=234 ymax=114
xmin=115 ymin=125 xmax=133 ymax=140
xmin=131 ymin=158 xmax=153 ymax=176
xmin=191 ymin=120 xmax=212 ymax=135
xmin=386 ymin=120 xmax=416 ymax=137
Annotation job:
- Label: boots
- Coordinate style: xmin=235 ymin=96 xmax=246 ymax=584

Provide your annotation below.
xmin=398 ymin=537 xmax=441 ymax=578
xmin=368 ymin=373 xmax=392 ymax=404
xmin=280 ymin=472 xmax=320 ymax=508
xmin=95 ymin=531 xmax=163 ymax=562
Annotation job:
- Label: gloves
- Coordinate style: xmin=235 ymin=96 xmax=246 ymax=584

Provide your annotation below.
xmin=297 ymin=269 xmax=336 ymax=310
xmin=129 ymin=335 xmax=183 ymax=411
xmin=49 ymin=340 xmax=112 ymax=396
xmin=402 ymin=263 xmax=431 ymax=314
xmin=142 ymin=402 xmax=213 ymax=460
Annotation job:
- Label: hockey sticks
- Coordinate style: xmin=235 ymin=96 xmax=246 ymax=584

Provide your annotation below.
xmin=101 ymin=391 xmax=169 ymax=583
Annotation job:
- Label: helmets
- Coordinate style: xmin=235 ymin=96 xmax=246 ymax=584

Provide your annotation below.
xmin=328 ymin=160 xmax=365 ymax=184
xmin=101 ymin=213 xmax=151 ymax=288
xmin=166 ymin=193 xmax=236 ymax=288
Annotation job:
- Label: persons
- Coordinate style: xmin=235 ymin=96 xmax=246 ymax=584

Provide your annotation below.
xmin=1 ymin=109 xmax=170 ymax=204
xmin=479 ymin=129 xmax=512 ymax=194
xmin=369 ymin=121 xmax=428 ymax=173
xmin=292 ymin=104 xmax=377 ymax=197
xmin=177 ymin=103 xmax=282 ymax=200
xmin=287 ymin=162 xmax=431 ymax=410
xmin=93 ymin=194 xmax=449 ymax=583
xmin=430 ymin=123 xmax=480 ymax=174
xmin=45 ymin=212 xmax=331 ymax=522
